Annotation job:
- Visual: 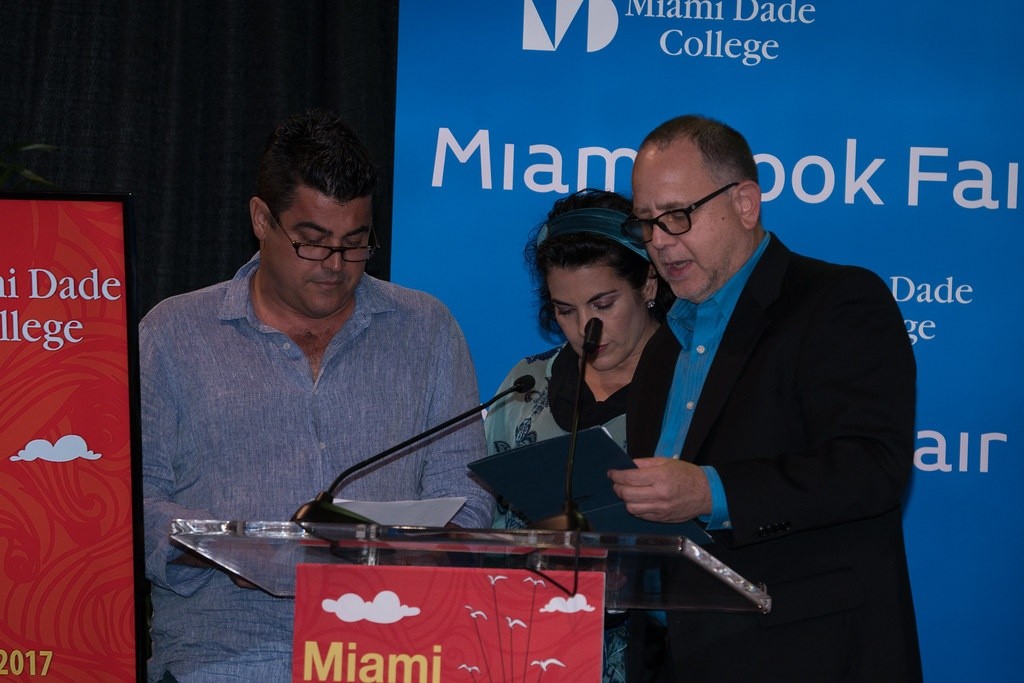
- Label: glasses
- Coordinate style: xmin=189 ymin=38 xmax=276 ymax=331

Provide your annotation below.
xmin=257 ymin=193 xmax=380 ymax=263
xmin=621 ymin=182 xmax=742 ymax=244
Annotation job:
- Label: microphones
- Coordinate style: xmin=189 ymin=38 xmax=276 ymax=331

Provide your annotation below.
xmin=290 ymin=375 xmax=536 ymax=525
xmin=530 ymin=317 xmax=605 ymax=530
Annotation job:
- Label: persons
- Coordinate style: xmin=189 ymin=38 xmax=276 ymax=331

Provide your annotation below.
xmin=482 ymin=187 xmax=678 ymax=683
xmin=136 ymin=104 xmax=494 ymax=683
xmin=606 ymin=112 xmax=926 ymax=683
